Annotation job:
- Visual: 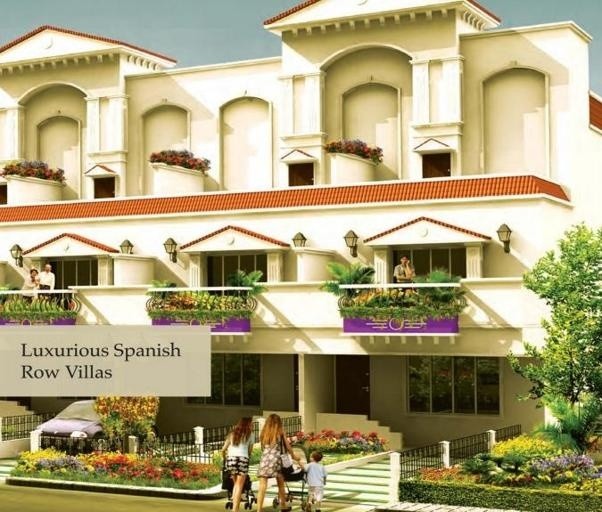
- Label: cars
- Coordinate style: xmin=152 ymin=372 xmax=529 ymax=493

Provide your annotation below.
xmin=35 ymin=400 xmax=158 ymax=452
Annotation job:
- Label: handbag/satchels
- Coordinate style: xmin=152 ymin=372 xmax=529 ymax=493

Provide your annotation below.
xmin=279 ymin=453 xmax=294 ymax=475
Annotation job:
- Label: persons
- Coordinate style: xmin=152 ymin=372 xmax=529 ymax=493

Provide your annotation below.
xmin=35 ymin=264 xmax=55 ymax=297
xmin=256 ymin=414 xmax=302 ymax=512
xmin=298 ymin=451 xmax=327 ymax=512
xmin=222 ymin=416 xmax=255 ymax=511
xmin=394 ymin=254 xmax=417 ymax=294
xmin=22 ymin=268 xmax=40 ymax=304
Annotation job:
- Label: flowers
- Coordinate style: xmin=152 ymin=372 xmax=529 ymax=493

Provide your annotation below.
xmin=150 ymin=150 xmax=210 ymax=176
xmin=0 ymin=159 xmax=66 ymax=184
xmin=324 ymin=139 xmax=383 ymax=166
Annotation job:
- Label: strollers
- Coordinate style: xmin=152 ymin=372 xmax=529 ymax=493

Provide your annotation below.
xmin=222 ymin=460 xmax=257 ymax=509
xmin=273 ymin=445 xmax=311 ymax=510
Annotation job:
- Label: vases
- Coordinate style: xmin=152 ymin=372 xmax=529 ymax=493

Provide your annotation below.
xmin=327 ymin=151 xmax=377 ymax=185
xmin=150 ymin=163 xmax=205 ymax=195
xmin=4 ymin=175 xmax=63 ymax=204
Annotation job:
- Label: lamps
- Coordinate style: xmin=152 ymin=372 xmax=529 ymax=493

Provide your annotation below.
xmin=163 ymin=237 xmax=177 ymax=262
xmin=9 ymin=244 xmax=23 ymax=266
xmin=343 ymin=230 xmax=360 ymax=257
xmin=292 ymin=232 xmax=305 ymax=248
xmin=120 ymin=240 xmax=133 ymax=255
xmin=496 ymin=224 xmax=512 ymax=252
